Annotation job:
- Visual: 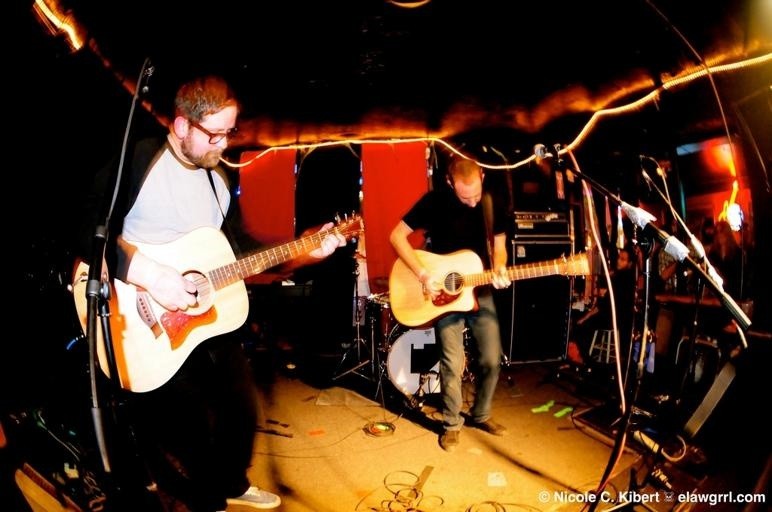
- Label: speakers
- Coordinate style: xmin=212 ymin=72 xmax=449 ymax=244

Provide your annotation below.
xmin=502 ymin=240 xmax=574 ymax=364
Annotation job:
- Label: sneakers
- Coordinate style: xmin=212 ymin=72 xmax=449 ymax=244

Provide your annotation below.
xmin=475 ymin=418 xmax=509 ymax=437
xmin=440 ymin=430 xmax=460 ymax=450
xmin=227 ymin=485 xmax=281 ymax=509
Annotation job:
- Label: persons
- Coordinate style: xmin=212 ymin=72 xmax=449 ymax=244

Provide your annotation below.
xmin=389 ymin=159 xmax=511 ymax=451
xmin=106 ymin=77 xmax=347 ymax=512
xmin=565 ymin=248 xmax=636 ymax=364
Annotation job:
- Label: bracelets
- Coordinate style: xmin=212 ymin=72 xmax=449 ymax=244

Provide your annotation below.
xmin=418 ymin=270 xmax=427 ymax=280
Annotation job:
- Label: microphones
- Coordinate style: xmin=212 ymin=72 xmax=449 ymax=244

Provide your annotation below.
xmin=607 ymin=150 xmax=650 ymax=160
xmin=633 ymin=430 xmax=664 ymax=455
xmin=141 ymin=65 xmax=156 ymax=95
xmin=682 ymin=334 xmax=719 ymax=349
xmin=533 ymin=143 xmax=563 ymax=166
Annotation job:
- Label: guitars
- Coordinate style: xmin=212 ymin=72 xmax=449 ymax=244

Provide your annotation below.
xmin=388 ymin=249 xmax=591 ymax=329
xmin=73 ymin=209 xmax=365 ymax=396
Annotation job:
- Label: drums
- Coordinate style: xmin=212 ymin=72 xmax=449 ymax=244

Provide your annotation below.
xmin=366 ymin=291 xmax=408 ymax=351
xmin=386 ymin=324 xmax=466 ymax=403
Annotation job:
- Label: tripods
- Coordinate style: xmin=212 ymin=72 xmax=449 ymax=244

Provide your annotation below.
xmin=332 ymin=273 xmax=388 ymax=409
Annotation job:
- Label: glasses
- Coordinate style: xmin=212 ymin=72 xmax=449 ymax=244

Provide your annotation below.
xmin=190 ymin=121 xmax=239 ymax=143
